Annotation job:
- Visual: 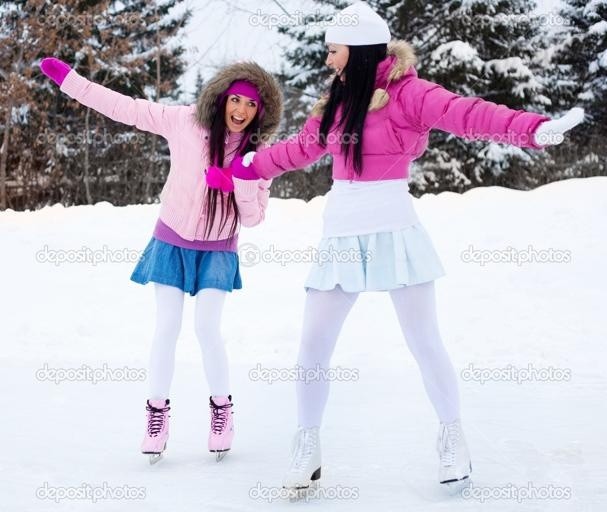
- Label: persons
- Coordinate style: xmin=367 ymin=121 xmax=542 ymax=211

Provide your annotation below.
xmin=39 ymin=57 xmax=284 ymax=455
xmin=241 ymin=1 xmax=585 ymax=490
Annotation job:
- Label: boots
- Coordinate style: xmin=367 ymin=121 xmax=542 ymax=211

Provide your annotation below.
xmin=437 ymin=420 xmax=471 ymax=484
xmin=283 ymin=424 xmax=323 ymax=488
xmin=207 ymin=395 xmax=234 ymax=453
xmin=141 ymin=400 xmax=171 ymax=455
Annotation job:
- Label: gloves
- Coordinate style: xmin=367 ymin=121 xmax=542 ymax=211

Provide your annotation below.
xmin=41 ymin=56 xmax=70 ymax=85
xmin=232 ymin=151 xmax=258 ymax=179
xmin=533 ymin=108 xmax=585 ymax=150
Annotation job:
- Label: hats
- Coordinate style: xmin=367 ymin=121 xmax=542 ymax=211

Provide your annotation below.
xmin=325 ymin=1 xmax=392 ymax=47
xmin=225 ymin=79 xmax=264 ymax=113
